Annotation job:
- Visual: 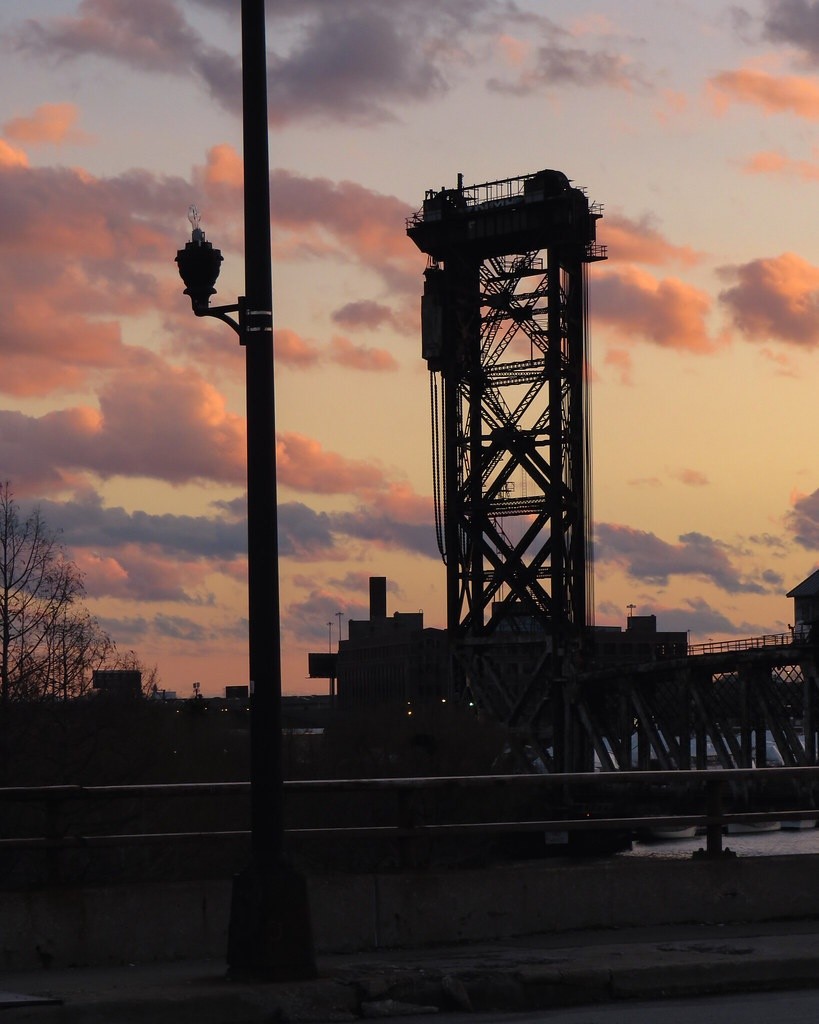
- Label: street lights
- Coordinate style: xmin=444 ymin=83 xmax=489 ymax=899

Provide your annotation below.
xmin=175 ymin=0 xmax=312 ymax=980
